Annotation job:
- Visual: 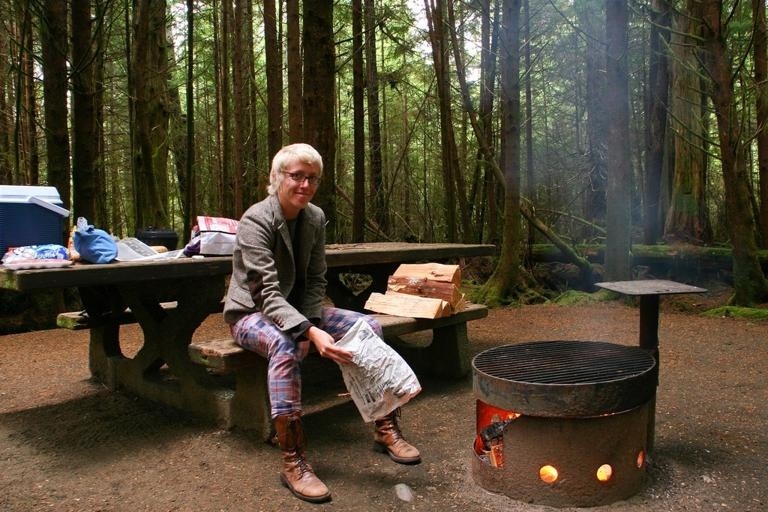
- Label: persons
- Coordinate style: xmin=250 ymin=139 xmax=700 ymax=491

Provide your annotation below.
xmin=222 ymin=141 xmax=423 ymax=503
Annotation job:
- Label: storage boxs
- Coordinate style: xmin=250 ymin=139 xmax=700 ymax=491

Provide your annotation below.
xmin=0 ymin=185 xmax=70 ymax=262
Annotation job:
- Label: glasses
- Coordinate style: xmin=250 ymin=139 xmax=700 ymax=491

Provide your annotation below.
xmin=279 ymin=170 xmax=320 ymax=186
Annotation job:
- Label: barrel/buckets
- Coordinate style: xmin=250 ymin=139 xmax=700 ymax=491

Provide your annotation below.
xmin=137 ymin=227 xmax=179 ymax=251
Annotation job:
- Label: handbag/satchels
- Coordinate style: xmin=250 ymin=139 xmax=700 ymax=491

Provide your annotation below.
xmin=73 ymin=222 xmax=118 ymax=264
xmin=176 ymin=214 xmax=240 ymax=261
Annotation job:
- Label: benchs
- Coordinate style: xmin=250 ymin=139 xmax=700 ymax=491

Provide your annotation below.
xmin=188 ymin=300 xmax=488 ymax=440
xmin=57 ymin=296 xmax=226 ymax=384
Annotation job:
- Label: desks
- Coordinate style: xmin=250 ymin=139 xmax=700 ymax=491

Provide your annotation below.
xmin=0 ymin=244 xmax=495 ymax=428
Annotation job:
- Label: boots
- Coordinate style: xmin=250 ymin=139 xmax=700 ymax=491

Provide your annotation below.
xmin=373 ymin=409 xmax=421 ymax=464
xmin=273 ymin=410 xmax=332 ymax=502
